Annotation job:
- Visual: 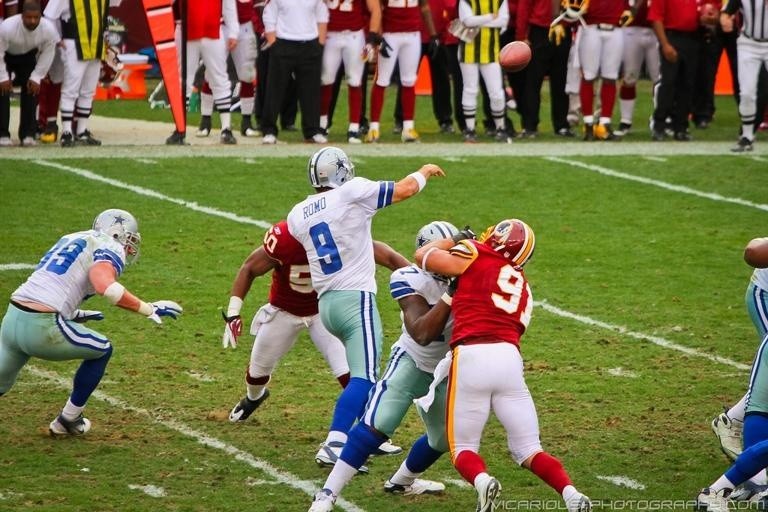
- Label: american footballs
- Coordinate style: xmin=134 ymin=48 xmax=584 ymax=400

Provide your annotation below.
xmin=499 ymin=41 xmax=532 ymax=69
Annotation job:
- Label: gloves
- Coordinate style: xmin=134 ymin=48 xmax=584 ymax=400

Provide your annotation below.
xmin=451 ymin=225 xmax=476 ymax=245
xmin=221 ymin=296 xmax=242 ymax=349
xmin=138 ymin=300 xmax=182 ymax=324
xmin=446 ymin=277 xmax=459 ymax=297
xmin=72 ymin=310 xmax=104 ymax=323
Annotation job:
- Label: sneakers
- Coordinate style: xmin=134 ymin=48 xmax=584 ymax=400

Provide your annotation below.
xmin=0 ymin=123 xmax=102 ymax=146
xmin=49 ymin=414 xmax=91 ymax=438
xmin=229 ymin=388 xmax=590 ymax=512
xmin=711 ymin=412 xmax=743 ymax=461
xmin=167 ymin=115 xmax=512 ymax=145
xmin=730 ymin=480 xmax=768 ymax=501
xmin=517 ymin=119 xmax=752 ymax=152
xmin=694 ymin=487 xmax=733 ymax=512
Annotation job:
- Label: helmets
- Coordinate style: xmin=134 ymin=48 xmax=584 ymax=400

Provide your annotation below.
xmin=416 ymin=221 xmax=459 ymax=249
xmin=308 ymin=147 xmax=354 ymax=190
xmin=92 ymin=209 xmax=141 ymax=266
xmin=479 ymin=219 xmax=535 ymax=267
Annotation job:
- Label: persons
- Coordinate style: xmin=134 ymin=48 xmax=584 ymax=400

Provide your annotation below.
xmin=0 ymin=206 xmax=184 ymax=440
xmin=303 ymin=221 xmax=458 ymax=512
xmin=285 ymin=144 xmax=448 ymax=476
xmin=0 ymin=0 xmax=767 ymax=153
xmin=220 ymin=219 xmax=413 ymax=454
xmin=695 ymin=235 xmax=767 ymax=512
xmin=413 ymin=217 xmax=595 ymax=512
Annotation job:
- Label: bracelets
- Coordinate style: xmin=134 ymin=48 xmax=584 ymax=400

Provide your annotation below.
xmin=406 ymin=172 xmax=427 ymax=196
xmin=441 ymin=292 xmax=453 ymax=306
